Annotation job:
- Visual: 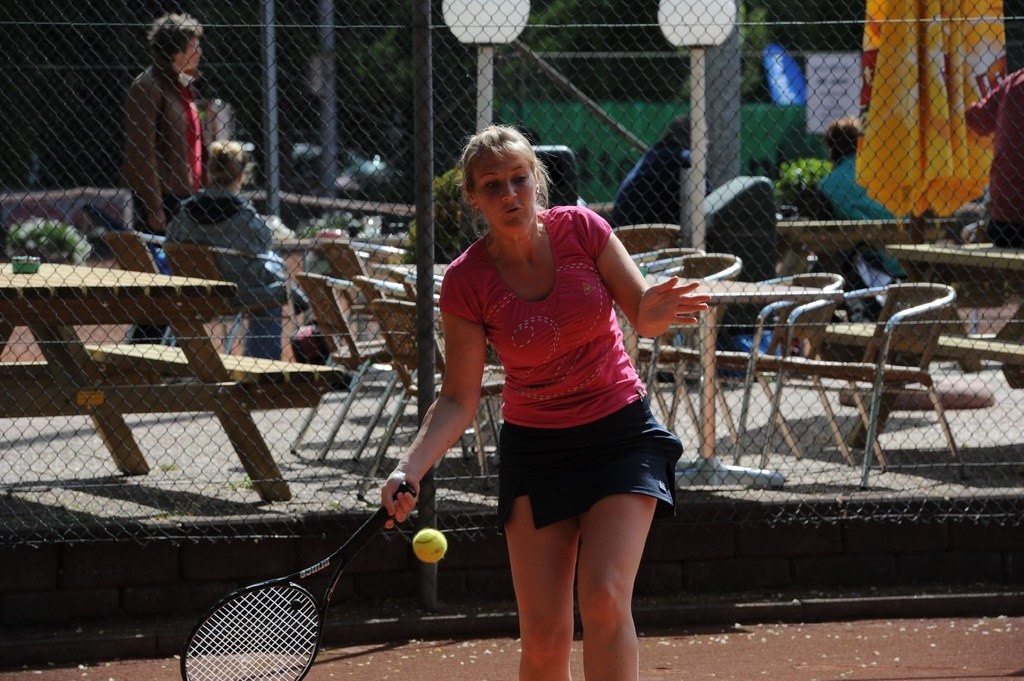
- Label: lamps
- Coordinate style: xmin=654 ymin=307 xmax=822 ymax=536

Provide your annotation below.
xmin=441 ymin=0 xmax=533 ymax=47
xmin=657 ymin=0 xmax=739 ymax=48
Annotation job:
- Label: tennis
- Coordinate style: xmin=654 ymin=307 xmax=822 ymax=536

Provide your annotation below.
xmin=412 ymin=527 xmax=448 ymax=564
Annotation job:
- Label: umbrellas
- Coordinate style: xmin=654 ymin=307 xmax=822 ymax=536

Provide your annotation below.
xmin=856 ymin=0 xmax=1006 ymax=280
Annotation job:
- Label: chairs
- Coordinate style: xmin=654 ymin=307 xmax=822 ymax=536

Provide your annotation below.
xmin=1 ymin=209 xmax=1024 ymax=498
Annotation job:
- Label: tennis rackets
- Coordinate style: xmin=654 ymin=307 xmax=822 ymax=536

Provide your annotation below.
xmin=179 ymin=479 xmax=418 ymax=681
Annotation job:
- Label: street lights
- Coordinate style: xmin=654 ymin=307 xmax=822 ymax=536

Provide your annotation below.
xmin=658 ymin=0 xmax=738 ymax=251
xmin=442 ymin=0 xmax=530 ymax=132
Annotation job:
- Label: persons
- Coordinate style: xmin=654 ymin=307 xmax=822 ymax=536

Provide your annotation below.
xmin=379 ymin=121 xmax=715 ymax=680
xmin=119 ymin=9 xmax=214 ymax=347
xmin=516 ymin=117 xmax=996 ymax=382
xmin=963 ymin=64 xmax=1024 ymax=393
xmin=162 ymin=140 xmax=312 ymax=386
